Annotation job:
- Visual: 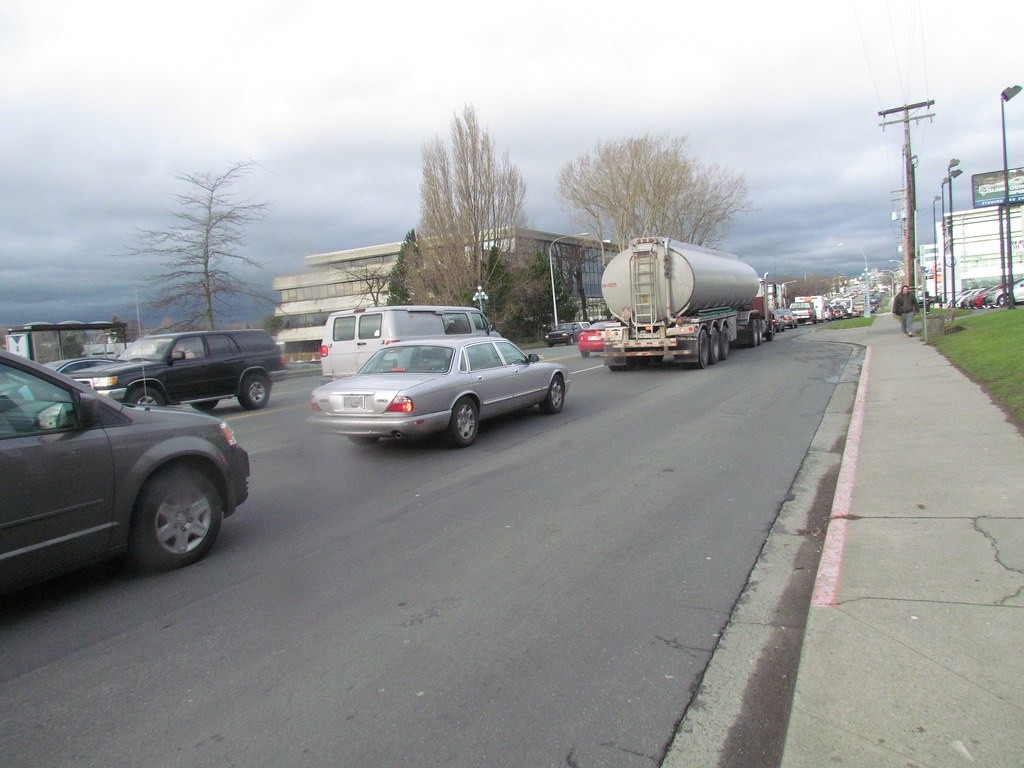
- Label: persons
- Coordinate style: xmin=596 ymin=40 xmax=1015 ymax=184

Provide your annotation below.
xmin=892 ymin=285 xmax=920 ymax=337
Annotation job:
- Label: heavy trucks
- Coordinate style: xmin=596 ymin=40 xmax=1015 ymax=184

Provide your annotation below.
xmin=599 ymin=234 xmax=777 ymax=373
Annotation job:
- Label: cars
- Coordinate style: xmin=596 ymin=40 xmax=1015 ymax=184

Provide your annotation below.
xmin=309 ymin=339 xmax=572 ymax=448
xmin=907 ymin=278 xmax=1024 ymax=310
xmin=0 ymin=349 xmax=254 ymax=596
xmin=768 ymin=289 xmax=880 ymax=331
xmin=0 ymin=356 xmax=113 ymax=403
xmin=578 ymin=321 xmax=622 ymax=358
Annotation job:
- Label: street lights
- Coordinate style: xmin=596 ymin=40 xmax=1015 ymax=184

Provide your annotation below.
xmin=870 ymin=258 xmax=906 ymax=314
xmin=999 ymin=85 xmax=1024 ymax=307
xmin=548 ymin=232 xmax=589 ymax=325
xmin=837 ymin=242 xmax=873 ymax=318
xmin=929 ymin=159 xmax=963 ymax=307
xmin=472 ymin=286 xmax=490 ymax=314
xmin=782 ymin=280 xmax=797 ymax=291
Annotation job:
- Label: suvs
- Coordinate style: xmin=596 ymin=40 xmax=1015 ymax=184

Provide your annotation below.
xmin=544 ymin=322 xmax=591 ymax=348
xmin=61 ymin=329 xmax=287 ymax=411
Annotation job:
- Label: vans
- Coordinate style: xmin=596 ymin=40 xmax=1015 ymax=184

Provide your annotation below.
xmin=318 ymin=306 xmax=502 ymax=386
